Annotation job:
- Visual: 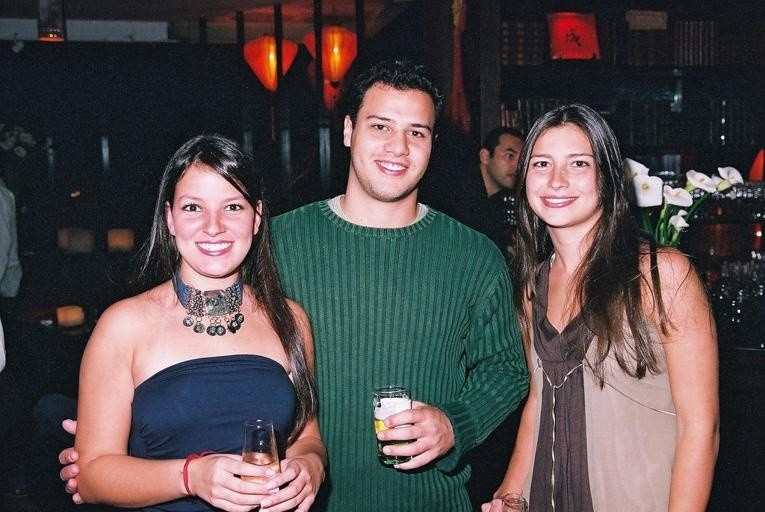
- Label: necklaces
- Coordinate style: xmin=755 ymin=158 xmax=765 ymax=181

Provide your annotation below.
xmin=168 ymin=266 xmax=246 ymax=335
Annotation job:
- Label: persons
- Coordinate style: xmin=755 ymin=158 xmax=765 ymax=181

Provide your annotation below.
xmin=72 ymin=132 xmax=328 ymax=512
xmin=479 ymin=101 xmax=724 ymax=512
xmin=57 ymin=59 xmax=530 ymax=512
xmin=0 ymin=179 xmax=24 ymax=373
xmin=423 ymin=124 xmax=525 ymax=243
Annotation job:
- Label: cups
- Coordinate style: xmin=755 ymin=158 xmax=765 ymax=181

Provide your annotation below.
xmin=504 ymin=493 xmax=525 ymax=511
xmin=372 ymin=385 xmax=413 ymax=467
xmin=239 ymin=420 xmax=281 ymax=491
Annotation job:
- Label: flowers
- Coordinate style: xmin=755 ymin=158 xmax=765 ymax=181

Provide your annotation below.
xmin=617 ymin=155 xmax=741 ymax=252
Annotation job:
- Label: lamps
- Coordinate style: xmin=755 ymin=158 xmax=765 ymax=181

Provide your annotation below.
xmin=33 ymin=0 xmax=68 ymax=43
xmin=237 ymin=16 xmax=297 ymax=94
xmin=299 ymin=3 xmax=354 ymax=86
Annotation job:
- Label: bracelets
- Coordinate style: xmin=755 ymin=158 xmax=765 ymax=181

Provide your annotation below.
xmin=183 ymin=449 xmax=205 ymax=500
